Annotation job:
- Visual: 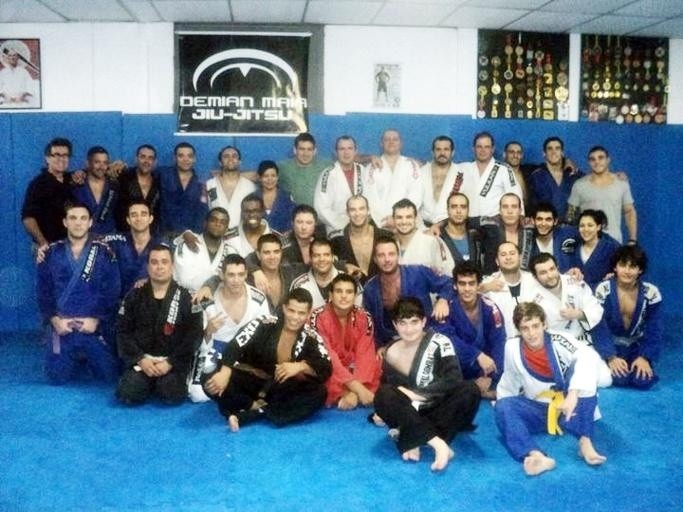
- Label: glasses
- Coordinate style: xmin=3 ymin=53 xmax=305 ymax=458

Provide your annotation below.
xmin=50 ymin=152 xmax=69 ymax=159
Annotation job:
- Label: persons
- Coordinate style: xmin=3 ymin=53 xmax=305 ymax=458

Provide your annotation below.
xmin=528 ymin=252 xmax=612 ymax=397
xmin=307 ymin=272 xmax=384 ymax=411
xmin=200 ymin=287 xmax=333 ymax=432
xmin=22 ymin=138 xmax=208 ymax=408
xmin=362 ymin=236 xmax=456 ymax=351
xmin=368 ymin=295 xmax=482 ymax=470
xmin=426 ymin=131 xmax=639 ymax=292
xmin=185 ymin=254 xmax=270 ymax=403
xmin=426 ymin=260 xmax=507 ymax=400
xmin=476 ymin=241 xmax=585 ymax=339
xmin=490 ymin=301 xmax=613 ymax=476
xmin=207 ymin=128 xmax=425 ymax=312
xmin=591 ymin=244 xmax=664 ymax=390
